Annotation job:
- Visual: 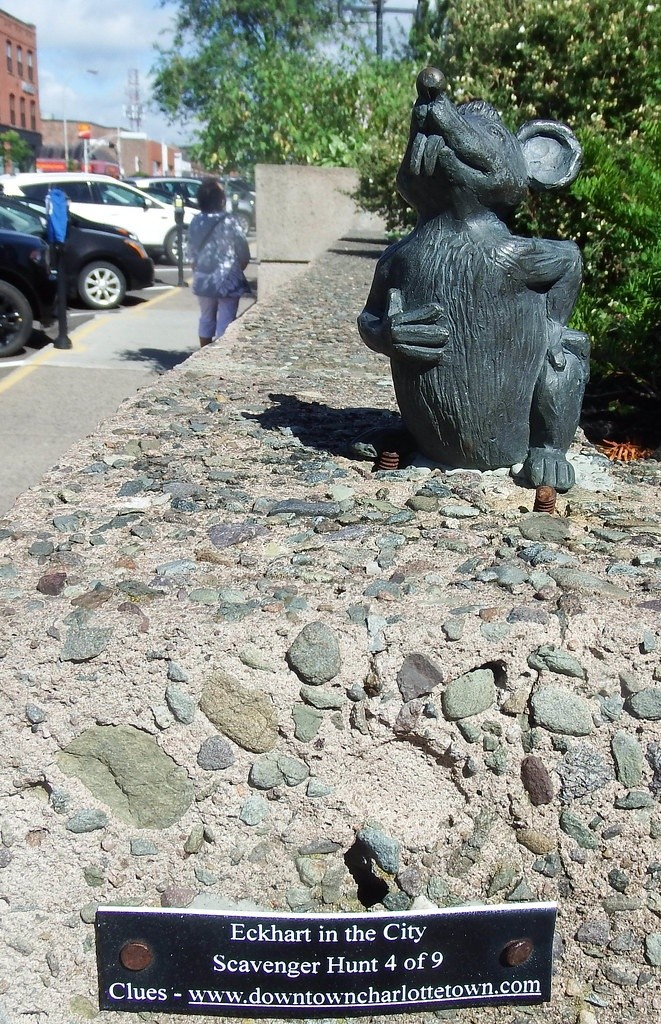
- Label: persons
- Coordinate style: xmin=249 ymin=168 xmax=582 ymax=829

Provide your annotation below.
xmin=186 ymin=180 xmax=250 ymax=347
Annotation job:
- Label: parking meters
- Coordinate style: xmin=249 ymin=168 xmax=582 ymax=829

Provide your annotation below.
xmin=174 ymin=194 xmax=189 ymax=289
xmin=47 ymin=189 xmax=73 ymax=349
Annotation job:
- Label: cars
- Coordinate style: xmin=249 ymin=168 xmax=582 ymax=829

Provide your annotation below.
xmin=0 ymin=172 xmax=203 ymax=268
xmin=107 ymin=178 xmax=256 ymax=238
xmin=0 ymin=195 xmax=155 ymax=312
xmin=88 ymin=68 xmax=124 ymax=179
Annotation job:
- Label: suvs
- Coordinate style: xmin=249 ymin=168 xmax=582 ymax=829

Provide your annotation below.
xmin=0 ymin=227 xmax=59 ymax=357
